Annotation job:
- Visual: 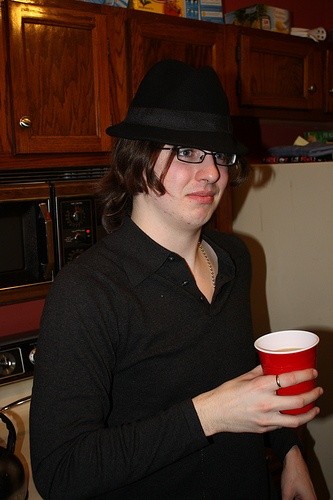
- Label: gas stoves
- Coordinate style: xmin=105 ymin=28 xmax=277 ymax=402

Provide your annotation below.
xmin=0 ymin=328 xmax=43 ymax=500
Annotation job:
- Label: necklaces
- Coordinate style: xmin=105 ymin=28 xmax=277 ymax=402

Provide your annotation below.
xmin=198 ymin=242 xmax=215 ymax=288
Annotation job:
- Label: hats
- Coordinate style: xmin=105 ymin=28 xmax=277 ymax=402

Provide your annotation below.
xmin=105 ymin=61 xmax=250 ymax=156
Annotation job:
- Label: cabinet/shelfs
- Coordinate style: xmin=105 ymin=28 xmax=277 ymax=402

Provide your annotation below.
xmin=0 ymin=0 xmax=333 ymax=170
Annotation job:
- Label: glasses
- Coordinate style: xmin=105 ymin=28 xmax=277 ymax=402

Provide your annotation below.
xmin=161 ymin=147 xmax=237 ymax=166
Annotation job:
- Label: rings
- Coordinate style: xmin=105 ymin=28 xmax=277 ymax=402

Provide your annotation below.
xmin=276 ymin=374 xmax=281 ymax=387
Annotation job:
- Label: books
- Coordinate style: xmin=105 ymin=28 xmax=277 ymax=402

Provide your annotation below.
xmin=260 ymin=143 xmax=333 ymax=163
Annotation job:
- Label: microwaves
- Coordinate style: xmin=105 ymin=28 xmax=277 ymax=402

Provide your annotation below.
xmin=0 ymin=169 xmax=122 ymax=304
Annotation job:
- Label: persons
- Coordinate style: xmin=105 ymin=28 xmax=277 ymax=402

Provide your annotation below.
xmin=29 ymin=60 xmax=323 ymax=500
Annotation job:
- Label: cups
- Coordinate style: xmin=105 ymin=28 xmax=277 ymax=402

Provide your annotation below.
xmin=253 ymin=330 xmax=319 ymax=417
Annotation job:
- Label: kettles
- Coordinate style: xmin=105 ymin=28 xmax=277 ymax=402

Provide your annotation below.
xmin=0 ymin=414 xmax=29 ymax=500
